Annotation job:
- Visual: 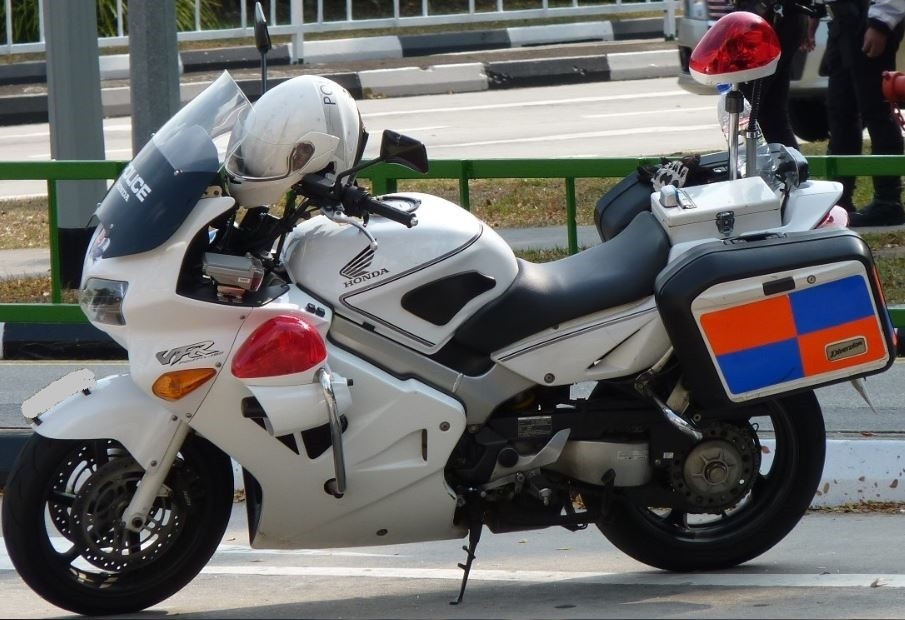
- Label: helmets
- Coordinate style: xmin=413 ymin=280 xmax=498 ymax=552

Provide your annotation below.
xmin=223 ymin=74 xmax=370 ymax=214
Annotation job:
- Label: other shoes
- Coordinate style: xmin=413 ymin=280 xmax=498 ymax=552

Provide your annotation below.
xmin=834 ymin=186 xmax=905 ymax=227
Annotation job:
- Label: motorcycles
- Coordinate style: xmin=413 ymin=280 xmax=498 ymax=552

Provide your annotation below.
xmin=2 ymin=2 xmax=897 ymax=619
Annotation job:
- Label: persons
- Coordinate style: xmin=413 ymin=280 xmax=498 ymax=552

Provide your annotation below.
xmin=805 ymin=0 xmax=905 ymax=227
xmin=724 ymin=0 xmax=827 ymax=179
